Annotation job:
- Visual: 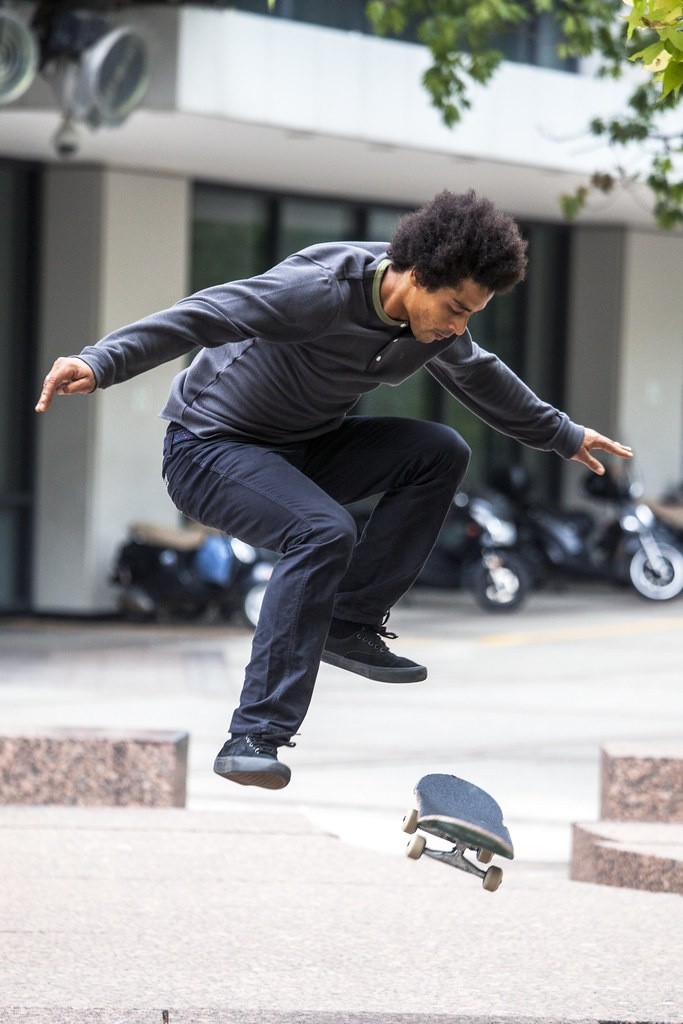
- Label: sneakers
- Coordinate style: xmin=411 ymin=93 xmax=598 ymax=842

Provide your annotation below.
xmin=213 ymin=731 xmax=300 ymax=790
xmin=319 ymin=610 xmax=427 ymax=684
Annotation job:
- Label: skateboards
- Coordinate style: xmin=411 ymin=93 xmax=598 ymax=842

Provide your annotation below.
xmin=401 ymin=772 xmax=514 ymax=893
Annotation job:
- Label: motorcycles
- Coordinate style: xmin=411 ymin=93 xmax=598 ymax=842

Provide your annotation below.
xmin=111 ymin=510 xmax=276 ymax=632
xmin=419 ymin=438 xmax=683 ymax=613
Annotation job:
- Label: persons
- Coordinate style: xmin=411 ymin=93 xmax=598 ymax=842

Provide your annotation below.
xmin=33 ymin=186 xmax=633 ymax=789
